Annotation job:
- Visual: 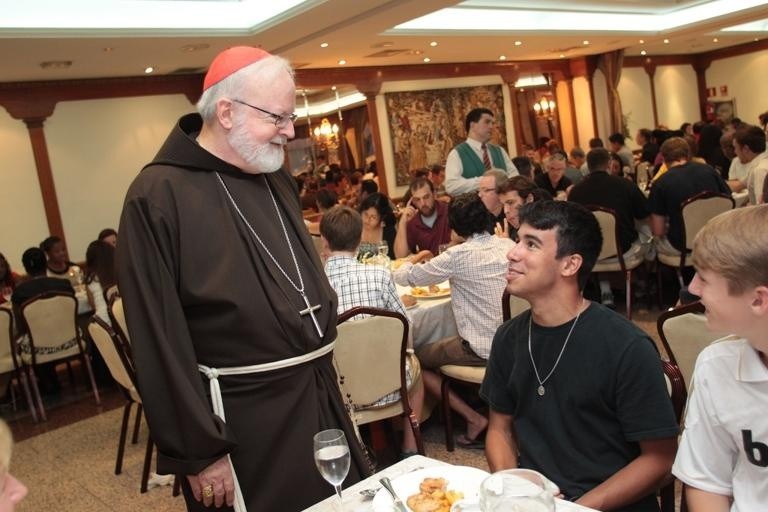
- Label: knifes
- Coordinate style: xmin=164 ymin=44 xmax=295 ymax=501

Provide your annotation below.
xmin=380 ymin=477 xmax=410 ymax=511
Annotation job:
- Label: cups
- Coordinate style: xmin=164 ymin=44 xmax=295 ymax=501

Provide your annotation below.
xmin=479 ymin=466 xmax=560 ymax=512
xmin=376 ymin=240 xmax=389 ymax=254
xmin=438 ymin=243 xmax=447 ymax=254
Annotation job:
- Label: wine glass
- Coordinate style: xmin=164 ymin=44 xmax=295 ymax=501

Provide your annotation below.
xmin=637 ymin=170 xmax=648 ymax=193
xmin=313 ymin=429 xmax=351 ymax=510
xmin=2 ymin=286 xmax=13 ymax=307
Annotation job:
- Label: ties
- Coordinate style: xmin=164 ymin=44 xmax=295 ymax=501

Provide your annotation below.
xmin=481 ymin=142 xmax=491 ymax=170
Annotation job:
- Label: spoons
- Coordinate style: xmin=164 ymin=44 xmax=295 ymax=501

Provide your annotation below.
xmin=359 ymin=466 xmax=427 ymax=497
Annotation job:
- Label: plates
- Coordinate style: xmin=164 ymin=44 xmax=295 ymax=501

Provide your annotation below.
xmin=372 ymin=466 xmax=487 ymax=511
xmin=411 ymin=290 xmax=450 ymax=298
xmin=404 ymin=303 xmax=420 ymax=311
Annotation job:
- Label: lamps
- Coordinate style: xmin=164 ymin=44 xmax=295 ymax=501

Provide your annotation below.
xmin=531 ymin=93 xmax=559 ymax=123
xmin=309 ymin=113 xmax=342 ymax=153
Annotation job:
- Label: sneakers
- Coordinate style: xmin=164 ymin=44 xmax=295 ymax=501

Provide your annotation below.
xmin=600 ymin=292 xmax=616 ymax=309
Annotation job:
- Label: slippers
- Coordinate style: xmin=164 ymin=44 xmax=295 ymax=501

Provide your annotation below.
xmin=456 ymin=434 xmax=486 ymax=449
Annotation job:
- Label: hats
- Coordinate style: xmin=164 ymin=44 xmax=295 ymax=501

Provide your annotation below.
xmin=202 ymin=46 xmax=271 ymax=92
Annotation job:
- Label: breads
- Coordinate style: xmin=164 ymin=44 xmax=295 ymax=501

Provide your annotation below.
xmin=408 ymin=477 xmax=462 ymax=512
xmin=429 ymin=285 xmax=440 ymax=293
xmin=400 ymin=294 xmax=417 ymax=307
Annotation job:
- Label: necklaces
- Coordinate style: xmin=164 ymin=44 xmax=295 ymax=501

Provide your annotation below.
xmin=213 ymin=171 xmax=324 ymax=337
xmin=528 ymin=299 xmax=585 ymax=395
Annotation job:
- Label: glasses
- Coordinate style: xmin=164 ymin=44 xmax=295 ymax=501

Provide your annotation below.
xmin=233 ymin=99 xmax=298 ymax=129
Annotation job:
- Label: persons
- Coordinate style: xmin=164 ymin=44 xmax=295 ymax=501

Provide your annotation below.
xmin=479 ymin=200 xmax=682 ymax=512
xmin=293 ymin=164 xmax=546 ymax=460
xmin=1 ymin=229 xmax=118 ymax=404
xmin=445 ymin=105 xmax=768 ymax=312
xmin=668 ymin=204 xmax=768 ymax=512
xmin=113 ymin=45 xmax=380 ymax=512
